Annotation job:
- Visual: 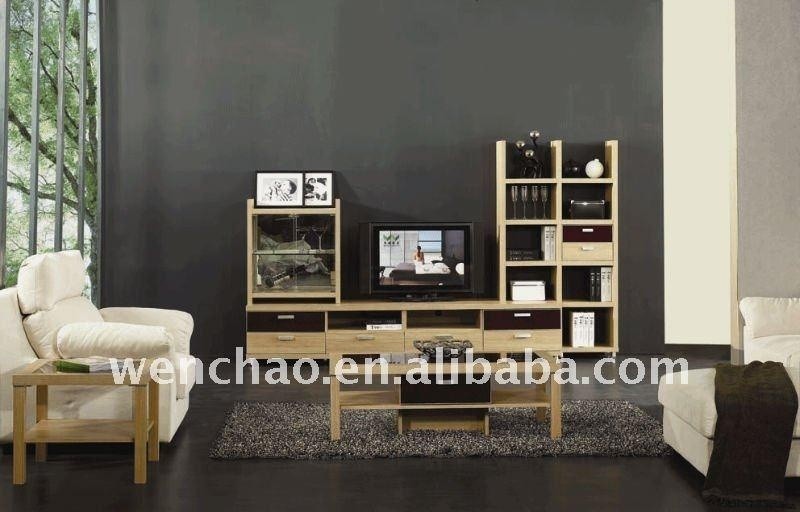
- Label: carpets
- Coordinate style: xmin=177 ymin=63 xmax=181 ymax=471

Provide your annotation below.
xmin=209 ymin=399 xmax=669 ymax=461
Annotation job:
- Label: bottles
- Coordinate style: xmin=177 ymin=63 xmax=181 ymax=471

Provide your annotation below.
xmin=266 ymin=264 xmax=305 ymax=287
xmin=589 ymin=272 xmax=600 ymax=302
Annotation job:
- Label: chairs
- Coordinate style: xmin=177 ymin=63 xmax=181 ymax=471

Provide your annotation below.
xmin=2 ymin=244 xmax=198 ymax=448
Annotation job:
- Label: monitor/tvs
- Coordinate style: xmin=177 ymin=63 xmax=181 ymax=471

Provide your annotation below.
xmin=368 ymin=219 xmax=474 ymax=302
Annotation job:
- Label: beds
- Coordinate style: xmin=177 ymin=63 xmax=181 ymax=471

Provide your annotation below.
xmin=328 ymin=349 xmax=561 ymax=445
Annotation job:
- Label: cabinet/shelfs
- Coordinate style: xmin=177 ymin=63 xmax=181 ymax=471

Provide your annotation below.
xmin=495 ymin=140 xmax=619 ymax=303
xmin=244 ymin=198 xmax=341 ymax=301
xmin=246 ymin=303 xmax=620 ymax=365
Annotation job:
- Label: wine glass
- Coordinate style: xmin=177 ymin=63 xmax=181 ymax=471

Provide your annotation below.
xmin=510 ymin=184 xmax=549 ymax=220
xmin=296 ymin=226 xmax=311 ymax=252
xmin=312 ymin=225 xmax=327 ymax=252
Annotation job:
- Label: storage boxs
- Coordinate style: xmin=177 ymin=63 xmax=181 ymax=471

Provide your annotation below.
xmin=562 ymin=226 xmax=613 ymax=261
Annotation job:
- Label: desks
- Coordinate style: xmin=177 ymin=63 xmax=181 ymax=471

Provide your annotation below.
xmin=11 ymin=354 xmax=161 ymax=486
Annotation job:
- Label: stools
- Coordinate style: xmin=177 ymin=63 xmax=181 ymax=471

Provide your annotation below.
xmin=657 ymin=367 xmax=800 ymax=480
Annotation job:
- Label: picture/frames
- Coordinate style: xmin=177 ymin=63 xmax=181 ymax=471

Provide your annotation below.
xmin=255 ymin=168 xmax=335 ymax=207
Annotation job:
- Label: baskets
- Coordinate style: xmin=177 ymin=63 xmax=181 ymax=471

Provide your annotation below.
xmin=414 ymin=339 xmax=472 ymax=360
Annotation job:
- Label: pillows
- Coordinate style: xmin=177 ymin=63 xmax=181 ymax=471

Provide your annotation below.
xmin=739 ymin=293 xmax=800 ymax=337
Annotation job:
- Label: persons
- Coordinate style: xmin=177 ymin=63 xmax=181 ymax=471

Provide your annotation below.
xmin=304 ymin=177 xmax=327 ymax=204
xmin=413 ymin=245 xmax=427 ymax=268
xmin=266 ymin=179 xmax=298 ymax=202
